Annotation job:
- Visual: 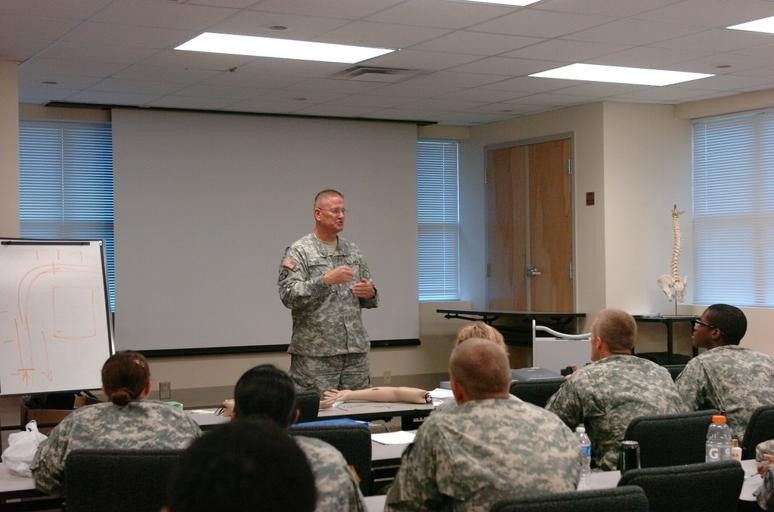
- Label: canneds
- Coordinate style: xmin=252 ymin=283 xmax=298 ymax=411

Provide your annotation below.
xmin=619 ymin=441 xmax=641 ymax=472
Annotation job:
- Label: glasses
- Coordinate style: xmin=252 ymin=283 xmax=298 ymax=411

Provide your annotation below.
xmin=694 ymin=318 xmax=723 ymax=335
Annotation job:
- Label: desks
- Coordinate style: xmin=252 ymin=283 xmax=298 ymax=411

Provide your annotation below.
xmin=630 ymin=314 xmax=701 ymax=362
xmin=436 ymin=308 xmax=586 ymax=350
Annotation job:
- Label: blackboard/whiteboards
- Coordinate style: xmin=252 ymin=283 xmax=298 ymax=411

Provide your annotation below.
xmin=0 ymin=238 xmax=115 ymax=395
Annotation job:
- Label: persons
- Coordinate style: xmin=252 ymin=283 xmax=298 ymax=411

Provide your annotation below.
xmin=675 ymin=303 xmax=773 ymax=444
xmin=753 ymin=435 xmax=774 ymax=511
xmin=225 ymin=361 xmax=369 ymax=512
xmin=161 ymin=419 xmax=318 ymax=511
xmin=380 ymin=336 xmax=584 ymax=511
xmin=30 ymin=350 xmax=205 ymax=497
xmin=319 ymin=319 xmax=506 ymax=405
xmin=544 ymin=307 xmax=686 ymax=472
xmin=277 ymin=188 xmax=381 ymax=400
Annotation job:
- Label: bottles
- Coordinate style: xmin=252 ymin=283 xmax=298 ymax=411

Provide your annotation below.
xmin=731 ymin=438 xmax=742 ymax=462
xmin=573 ymin=425 xmax=593 ymax=489
xmin=704 ymin=414 xmax=732 ymax=463
